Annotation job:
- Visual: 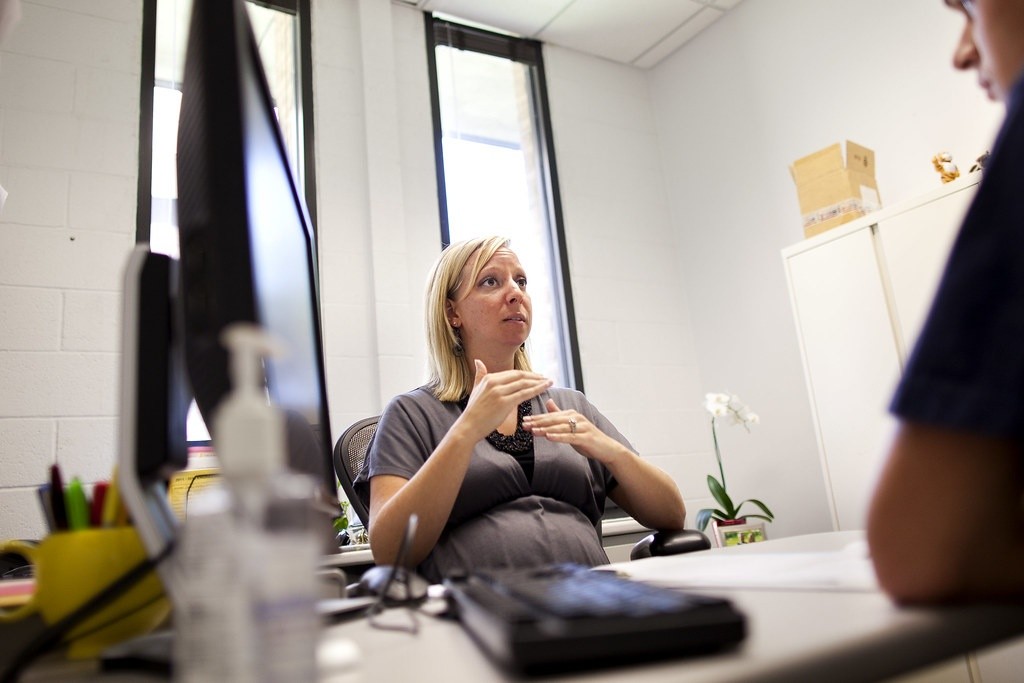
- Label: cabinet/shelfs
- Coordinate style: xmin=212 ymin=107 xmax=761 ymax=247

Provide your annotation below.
xmin=781 ymin=166 xmax=984 ymax=535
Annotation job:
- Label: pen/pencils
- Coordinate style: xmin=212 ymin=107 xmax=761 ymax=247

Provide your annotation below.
xmin=50 ymin=464 xmax=67 ymax=532
xmin=37 ymin=484 xmax=57 ymax=531
xmin=92 ymin=481 xmax=109 ymax=527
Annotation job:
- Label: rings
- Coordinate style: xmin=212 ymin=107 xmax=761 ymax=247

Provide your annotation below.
xmin=568 ymin=419 xmax=577 ymax=433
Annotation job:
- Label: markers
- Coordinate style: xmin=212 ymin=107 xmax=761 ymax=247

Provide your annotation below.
xmin=64 ymin=480 xmax=90 ymax=529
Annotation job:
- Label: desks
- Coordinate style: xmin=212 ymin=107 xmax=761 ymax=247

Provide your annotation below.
xmin=316 ymin=516 xmax=1024 ymax=682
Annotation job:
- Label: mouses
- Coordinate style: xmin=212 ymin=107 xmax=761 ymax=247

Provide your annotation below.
xmin=358 ymin=564 xmax=429 ymax=607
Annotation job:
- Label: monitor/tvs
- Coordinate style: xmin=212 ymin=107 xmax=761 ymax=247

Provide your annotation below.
xmin=97 ymin=0 xmax=381 ymax=677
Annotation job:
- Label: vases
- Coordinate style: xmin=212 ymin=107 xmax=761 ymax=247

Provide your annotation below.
xmin=716 ymin=519 xmax=746 ymax=527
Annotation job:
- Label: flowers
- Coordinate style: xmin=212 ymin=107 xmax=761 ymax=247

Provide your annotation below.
xmin=694 ymin=393 xmax=774 ymax=533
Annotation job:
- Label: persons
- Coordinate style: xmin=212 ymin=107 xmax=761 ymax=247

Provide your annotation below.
xmin=866 ymin=0 xmax=1023 ymax=611
xmin=353 ymin=233 xmax=685 ymax=585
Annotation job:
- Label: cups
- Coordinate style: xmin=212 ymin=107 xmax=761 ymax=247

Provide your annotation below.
xmin=0 ymin=528 xmax=174 ymax=659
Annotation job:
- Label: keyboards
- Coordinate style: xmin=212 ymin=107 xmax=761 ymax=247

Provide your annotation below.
xmin=443 ymin=562 xmax=751 ymax=681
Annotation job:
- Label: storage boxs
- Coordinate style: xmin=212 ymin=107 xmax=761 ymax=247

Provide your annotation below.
xmin=789 ymin=140 xmax=884 ymax=240
